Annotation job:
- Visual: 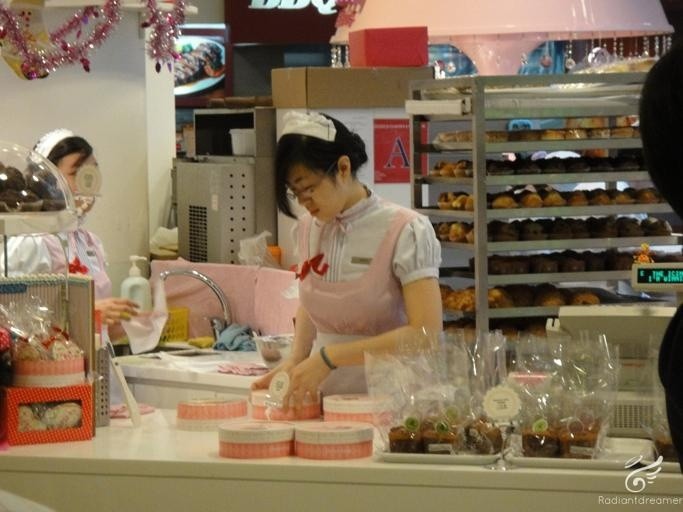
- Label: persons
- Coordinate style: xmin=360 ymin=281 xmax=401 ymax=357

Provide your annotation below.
xmin=249 ymin=109 xmax=446 ymax=419
xmin=0 ymin=128 xmax=143 ymax=403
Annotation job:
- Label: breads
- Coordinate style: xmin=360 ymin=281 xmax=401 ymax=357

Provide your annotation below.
xmin=429 ymin=113 xmax=683 ymax=336
xmin=389 ymin=415 xmax=599 ymax=458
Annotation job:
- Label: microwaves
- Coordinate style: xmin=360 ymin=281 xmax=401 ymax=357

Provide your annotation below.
xmin=192 ymin=106 xmax=276 ymax=163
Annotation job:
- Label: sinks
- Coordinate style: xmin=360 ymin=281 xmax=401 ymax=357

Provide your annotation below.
xmin=112 ymin=343 xmax=191 ymax=358
xmin=173 ymin=351 xmax=218 ymax=358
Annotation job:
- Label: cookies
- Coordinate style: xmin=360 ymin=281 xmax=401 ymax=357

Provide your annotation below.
xmin=15 ymin=339 xmax=81 ymax=431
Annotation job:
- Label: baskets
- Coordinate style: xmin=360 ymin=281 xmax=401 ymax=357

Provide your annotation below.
xmin=119 ymin=305 xmax=190 ymax=348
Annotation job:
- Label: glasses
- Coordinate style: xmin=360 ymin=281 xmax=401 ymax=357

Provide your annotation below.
xmin=284 ymin=159 xmax=336 ymax=203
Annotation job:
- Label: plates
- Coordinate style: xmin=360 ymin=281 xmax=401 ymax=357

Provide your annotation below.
xmin=174 ymin=35 xmax=226 ymax=96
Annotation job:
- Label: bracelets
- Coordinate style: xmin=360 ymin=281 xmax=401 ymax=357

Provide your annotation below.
xmin=319 ymin=346 xmax=337 ymax=370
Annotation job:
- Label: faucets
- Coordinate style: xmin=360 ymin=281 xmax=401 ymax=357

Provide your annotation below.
xmin=160 ymin=267 xmax=233 ymax=339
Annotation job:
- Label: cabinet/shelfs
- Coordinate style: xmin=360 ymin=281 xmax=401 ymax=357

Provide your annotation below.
xmin=407 ymin=70 xmax=683 ymax=353
